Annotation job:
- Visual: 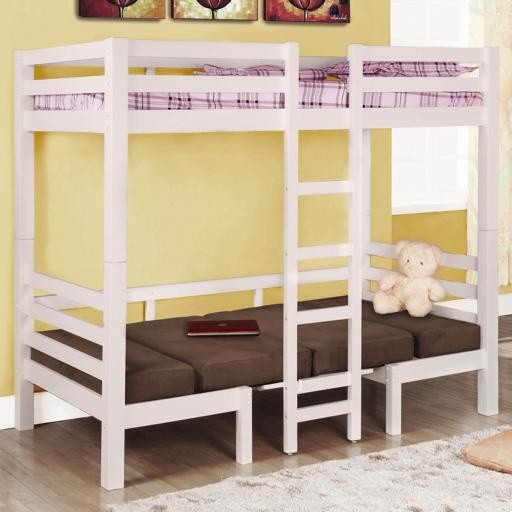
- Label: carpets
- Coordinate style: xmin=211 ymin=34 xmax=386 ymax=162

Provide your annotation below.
xmin=434 ymin=292 xmax=511 ymax=359
xmin=109 ymin=422 xmax=512 ymax=510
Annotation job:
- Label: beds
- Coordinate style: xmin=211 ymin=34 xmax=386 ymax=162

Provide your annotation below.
xmin=14 ymin=33 xmax=501 ymax=495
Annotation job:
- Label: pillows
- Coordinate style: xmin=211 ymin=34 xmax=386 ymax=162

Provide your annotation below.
xmin=461 ymin=429 xmax=512 ymax=474
xmin=319 ymin=58 xmax=478 ymax=78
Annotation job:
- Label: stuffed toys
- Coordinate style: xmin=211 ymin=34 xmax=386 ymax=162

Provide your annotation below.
xmin=370 ymin=238 xmax=447 ymax=320
